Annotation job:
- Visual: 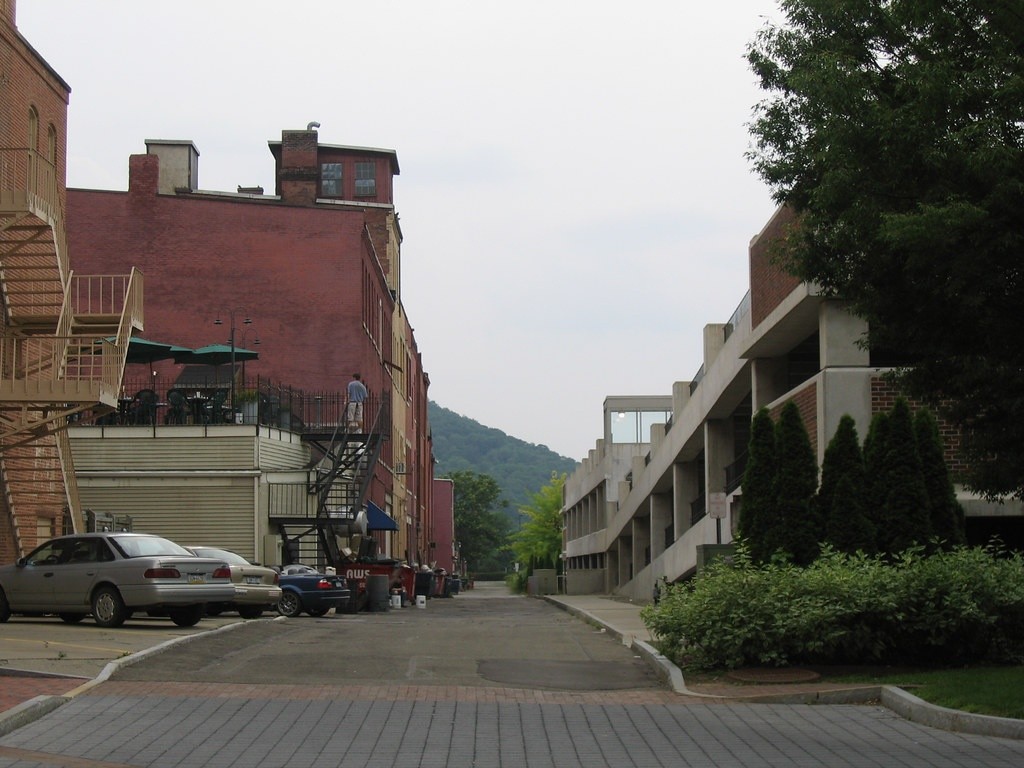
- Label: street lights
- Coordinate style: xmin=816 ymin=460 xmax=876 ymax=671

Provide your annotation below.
xmin=225 ymin=326 xmax=263 ymax=393
xmin=518 ymin=514 xmax=524 ymax=534
xmin=213 ymin=304 xmax=251 ymax=424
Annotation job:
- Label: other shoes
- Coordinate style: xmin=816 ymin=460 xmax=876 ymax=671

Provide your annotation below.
xmin=353 ymin=428 xmax=362 ymax=434
xmin=342 ymin=428 xmax=349 ymax=434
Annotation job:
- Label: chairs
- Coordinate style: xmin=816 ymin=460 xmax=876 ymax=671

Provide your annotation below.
xmin=95 ymin=388 xmax=237 ymax=425
xmin=67 ymin=549 xmax=89 ymax=564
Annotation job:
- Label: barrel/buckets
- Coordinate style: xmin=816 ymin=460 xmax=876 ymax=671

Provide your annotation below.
xmin=335 ymin=578 xmax=358 ymax=614
xmin=416 ymin=595 xmax=426 ymax=609
xmin=235 ymin=413 xmax=243 ymax=424
xmin=392 ymin=596 xmax=401 ymax=608
xmin=366 ymin=574 xmax=389 ymax=612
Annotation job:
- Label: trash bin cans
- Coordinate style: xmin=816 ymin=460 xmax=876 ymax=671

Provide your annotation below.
xmin=451 ymin=579 xmax=460 ymax=595
xmin=414 ymin=572 xmax=433 ymax=600
xmin=366 ymin=574 xmax=389 ymax=613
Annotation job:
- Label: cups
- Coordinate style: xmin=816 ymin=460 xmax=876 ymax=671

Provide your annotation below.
xmin=197 ymin=392 xmax=200 ymax=398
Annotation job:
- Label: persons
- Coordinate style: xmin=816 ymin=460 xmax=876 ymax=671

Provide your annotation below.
xmin=343 ymin=373 xmax=369 ymax=434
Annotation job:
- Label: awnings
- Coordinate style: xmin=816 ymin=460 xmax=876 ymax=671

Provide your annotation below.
xmin=367 ymin=500 xmax=400 ymax=531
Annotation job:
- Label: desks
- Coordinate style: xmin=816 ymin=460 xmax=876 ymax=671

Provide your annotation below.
xmin=117 ymin=397 xmax=134 ymax=424
xmin=208 ymin=404 xmax=230 ymax=424
xmin=183 ymin=396 xmax=210 ymax=426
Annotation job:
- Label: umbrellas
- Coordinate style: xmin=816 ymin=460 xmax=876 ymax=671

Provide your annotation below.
xmin=76 ymin=334 xmax=259 ymax=424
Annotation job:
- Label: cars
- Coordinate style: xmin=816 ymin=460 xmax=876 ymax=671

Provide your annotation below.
xmin=0 ymin=531 xmax=235 ymax=627
xmin=148 ymin=546 xmax=283 ymax=619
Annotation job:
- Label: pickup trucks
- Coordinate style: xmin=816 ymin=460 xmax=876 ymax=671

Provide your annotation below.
xmin=255 ymin=564 xmax=353 ymax=617
